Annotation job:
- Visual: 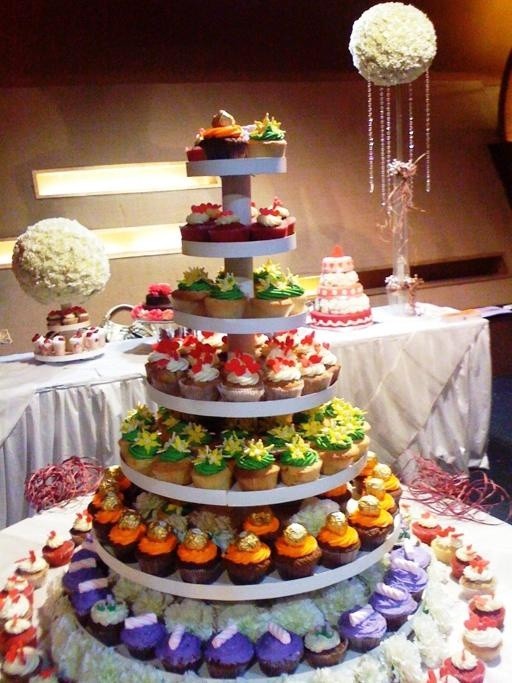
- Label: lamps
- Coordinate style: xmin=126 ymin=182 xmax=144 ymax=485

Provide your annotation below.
xmin=32 ymin=160 xmax=222 ymax=200
xmin=0 ymin=225 xmax=183 ymax=267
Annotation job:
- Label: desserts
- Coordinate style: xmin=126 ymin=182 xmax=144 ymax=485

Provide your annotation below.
xmin=87 ymin=452 xmax=403 ymax=585
xmin=61 ymin=539 xmax=431 ymax=678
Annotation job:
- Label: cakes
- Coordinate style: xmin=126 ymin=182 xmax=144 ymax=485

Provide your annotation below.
xmin=308 ymin=246 xmax=371 ymax=329
xmin=186 ymin=112 xmax=287 ymax=160
xmin=172 ymin=260 xmax=307 ymax=316
xmin=180 ymin=196 xmax=296 ymax=242
xmin=145 ymin=330 xmax=341 ymax=403
xmin=0 ymin=508 xmax=93 ymax=683
xmin=412 ymin=512 xmax=505 ymax=682
xmin=118 ymin=402 xmax=371 ymax=491
xmin=31 ymin=307 xmax=107 ymax=357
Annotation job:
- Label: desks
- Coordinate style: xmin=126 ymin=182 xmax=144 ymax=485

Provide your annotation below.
xmin=0 ymin=482 xmax=512 ymax=683
